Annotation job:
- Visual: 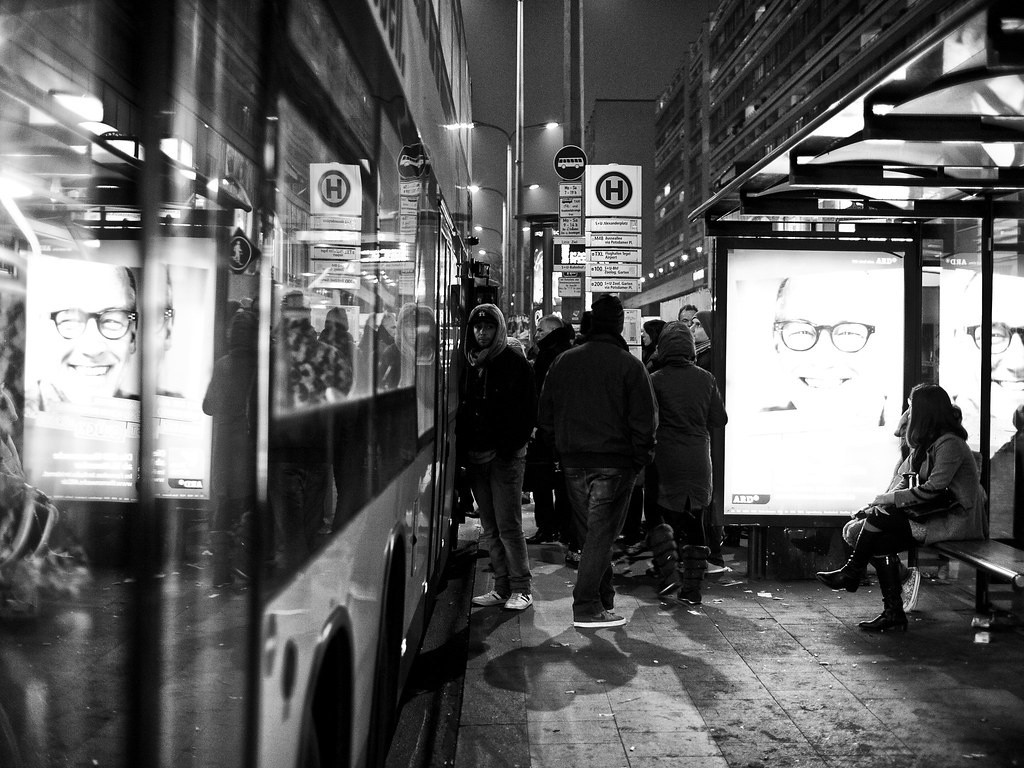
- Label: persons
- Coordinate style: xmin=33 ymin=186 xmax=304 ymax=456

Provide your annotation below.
xmin=536 ymin=294 xmax=660 ymax=629
xmin=454 ymin=303 xmax=749 ymax=611
xmin=33 ymin=258 xmax=141 ymax=414
xmin=952 ymin=266 xmax=1024 ymax=443
xmin=141 ymin=260 xmax=187 ymax=401
xmin=200 ymin=286 xmax=439 ymax=598
xmin=758 ymin=272 xmax=893 ymax=434
xmin=815 ymin=382 xmax=988 ymax=631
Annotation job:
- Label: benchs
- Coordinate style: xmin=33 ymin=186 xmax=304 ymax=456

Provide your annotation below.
xmin=904 ymin=535 xmax=1024 ymax=623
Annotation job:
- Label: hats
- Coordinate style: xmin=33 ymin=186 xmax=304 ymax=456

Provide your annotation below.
xmin=691 ymin=310 xmax=713 ymax=338
xmin=326 ymin=307 xmax=349 ymax=330
xmin=469 ymin=310 xmax=498 ymax=325
xmin=281 ymin=290 xmax=311 ymax=319
xmin=591 ymin=294 xmax=624 ymax=334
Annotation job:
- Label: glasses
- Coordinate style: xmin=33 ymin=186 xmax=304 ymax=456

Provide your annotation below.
xmin=50 ymin=307 xmax=137 ymax=340
xmin=774 ymin=322 xmax=873 ymax=352
xmin=954 ymin=321 xmax=1024 ymax=354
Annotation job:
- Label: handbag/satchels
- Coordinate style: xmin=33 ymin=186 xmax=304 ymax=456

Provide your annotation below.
xmin=903 ymin=494 xmax=960 ymax=524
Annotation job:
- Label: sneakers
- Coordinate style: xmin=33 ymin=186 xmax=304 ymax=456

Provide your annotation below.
xmin=505 ymin=592 xmax=533 ymax=609
xmin=574 ymin=610 xmax=627 ymax=627
xmin=902 ymin=567 xmax=920 ymax=612
xmin=472 ymin=590 xmax=506 ymax=605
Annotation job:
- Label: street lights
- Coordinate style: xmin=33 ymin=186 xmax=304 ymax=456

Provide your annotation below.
xmin=472 ymin=116 xmax=562 ymax=321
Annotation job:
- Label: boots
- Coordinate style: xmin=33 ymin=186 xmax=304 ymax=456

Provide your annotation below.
xmin=859 ymin=565 xmax=908 ymax=631
xmin=677 ymin=545 xmax=711 ymax=604
xmin=650 ymin=525 xmax=680 ymax=595
xmin=816 ymin=527 xmax=882 ymax=593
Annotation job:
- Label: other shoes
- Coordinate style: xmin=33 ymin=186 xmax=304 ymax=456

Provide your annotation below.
xmin=525 ymin=527 xmax=559 ymax=544
xmin=186 ymin=561 xmax=207 ymax=569
xmin=214 ymin=575 xmax=235 ymax=588
xmin=233 ymin=563 xmax=251 ymax=580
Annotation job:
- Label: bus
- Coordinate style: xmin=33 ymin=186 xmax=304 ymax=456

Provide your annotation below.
xmin=0 ymin=1 xmax=478 ymax=768
